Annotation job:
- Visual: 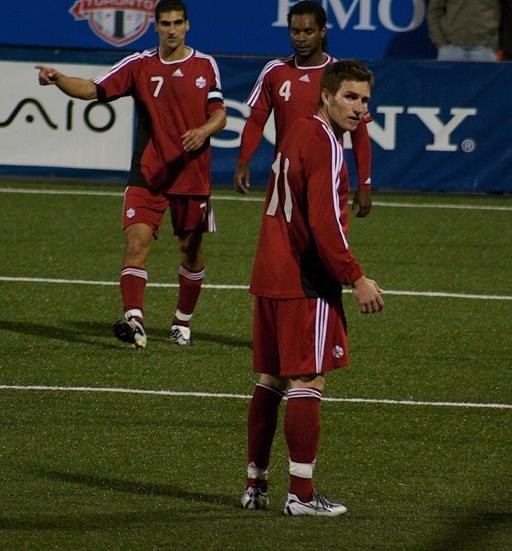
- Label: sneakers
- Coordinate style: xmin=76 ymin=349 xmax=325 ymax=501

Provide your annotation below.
xmin=282 ymin=490 xmax=347 ymax=519
xmin=241 ymin=475 xmax=275 ymax=511
xmin=168 ymin=322 xmax=194 ymax=349
xmin=111 ymin=313 xmax=148 ymax=351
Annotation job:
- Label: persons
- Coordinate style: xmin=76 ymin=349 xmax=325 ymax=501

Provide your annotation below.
xmin=426 ymin=1 xmax=505 ymax=63
xmin=239 ymin=61 xmax=384 ymax=517
xmin=233 ymin=1 xmax=375 ymax=221
xmin=34 ymin=0 xmax=227 ymax=350
